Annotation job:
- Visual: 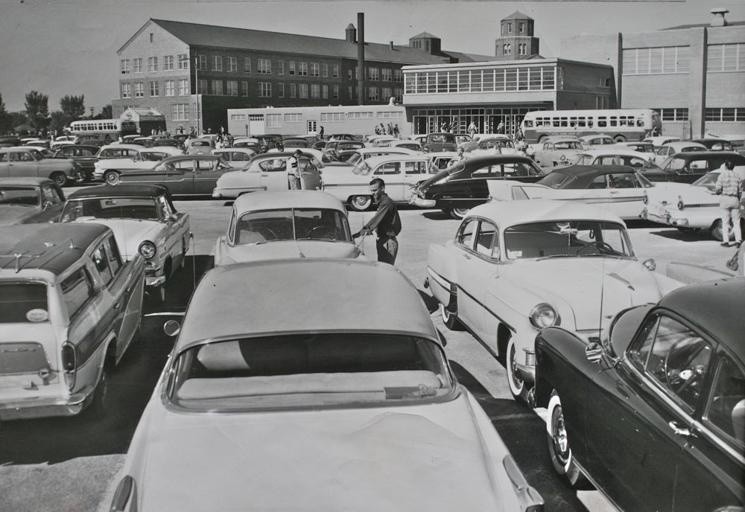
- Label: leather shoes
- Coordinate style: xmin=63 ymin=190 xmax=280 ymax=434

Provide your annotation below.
xmin=735 ymin=242 xmax=740 ymax=248
xmin=720 ymin=242 xmax=730 ymax=247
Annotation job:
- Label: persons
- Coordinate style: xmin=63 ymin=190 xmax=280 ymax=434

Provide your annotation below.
xmin=457 ymin=147 xmax=465 ymax=163
xmin=715 ymin=161 xmax=744 ymax=248
xmin=150 ymin=128 xmax=168 ymax=136
xmin=215 ymin=136 xmax=224 ymax=149
xmin=497 ymin=119 xmax=505 ymax=133
xmin=226 ymin=133 xmax=235 ymax=148
xmin=352 ymin=177 xmax=402 ymax=265
xmin=374 ymin=121 xmax=400 ymax=137
xmin=318 ymin=125 xmax=325 ymax=140
xmin=467 ymin=121 xmax=479 ymax=139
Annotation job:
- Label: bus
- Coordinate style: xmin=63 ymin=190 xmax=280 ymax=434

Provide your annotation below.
xmin=516 ymin=108 xmax=669 ymax=147
xmin=66 ymin=119 xmax=137 ymax=139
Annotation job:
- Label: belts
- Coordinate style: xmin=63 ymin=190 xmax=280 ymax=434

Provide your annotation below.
xmin=721 ymin=193 xmax=739 ymax=197
